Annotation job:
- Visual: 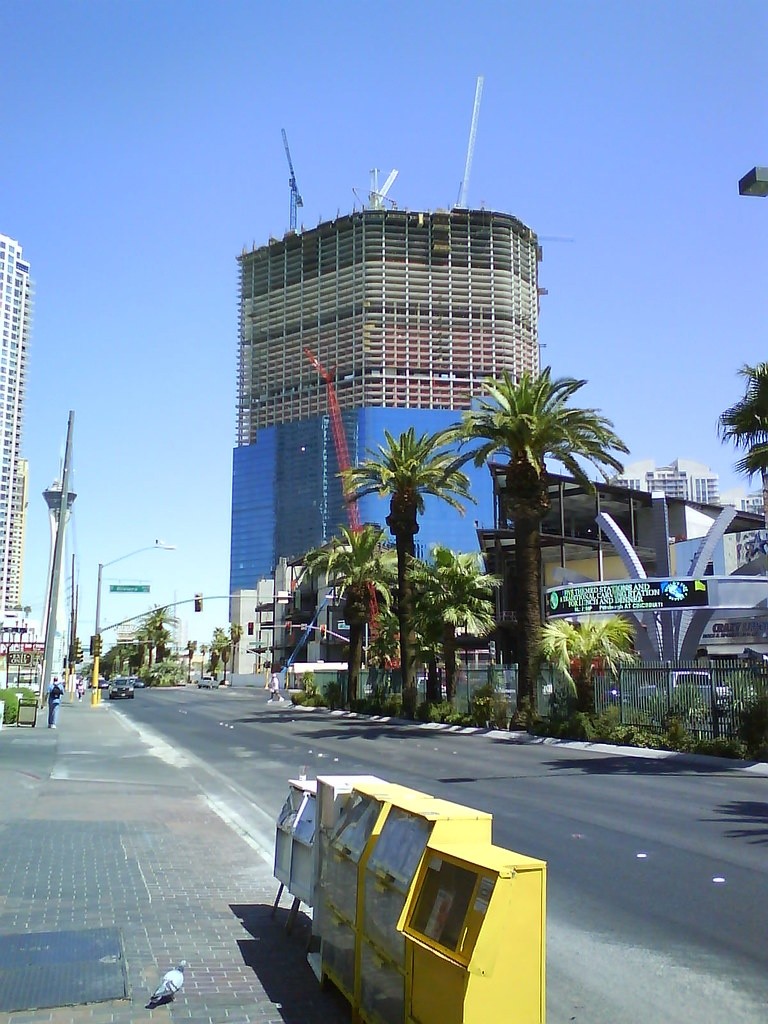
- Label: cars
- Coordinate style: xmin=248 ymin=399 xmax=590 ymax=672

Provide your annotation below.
xmin=197 ymin=676 xmax=219 ymax=690
xmin=89 ymin=678 xmax=108 ymax=689
xmin=130 ymin=678 xmax=146 ymax=689
xmin=655 ymin=670 xmax=714 ymax=717
xmin=107 ymin=677 xmax=135 ymax=700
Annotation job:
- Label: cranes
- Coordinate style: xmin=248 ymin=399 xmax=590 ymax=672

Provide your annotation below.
xmin=300 ymin=343 xmax=405 ymax=670
xmin=350 ymin=166 xmax=401 ymax=212
xmin=279 ymin=126 xmax=304 ymax=234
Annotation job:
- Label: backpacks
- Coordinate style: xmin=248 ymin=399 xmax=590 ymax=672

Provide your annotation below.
xmin=50 ymin=683 xmax=60 ymax=698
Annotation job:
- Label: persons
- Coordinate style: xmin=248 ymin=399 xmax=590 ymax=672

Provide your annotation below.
xmin=267 ymin=674 xmax=284 ymax=702
xmin=78 ymin=678 xmax=86 ymax=703
xmin=45 ymin=676 xmax=64 ymax=728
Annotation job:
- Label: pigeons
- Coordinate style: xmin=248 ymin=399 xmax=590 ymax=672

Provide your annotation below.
xmin=150 ymin=965 xmax=185 ymax=1004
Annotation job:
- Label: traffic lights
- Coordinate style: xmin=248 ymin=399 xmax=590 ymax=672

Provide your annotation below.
xmin=248 ymin=622 xmax=253 ymax=635
xmin=319 ymin=624 xmax=326 ymax=640
xmin=94 ymin=634 xmax=103 ymax=656
xmin=76 ymin=638 xmax=84 ymax=663
xmin=285 ymin=621 xmax=291 ymax=635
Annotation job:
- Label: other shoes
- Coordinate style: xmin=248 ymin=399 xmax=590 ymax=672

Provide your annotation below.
xmin=278 ymin=697 xmax=284 ymax=701
xmin=48 ymin=723 xmax=55 ymax=728
xmin=268 ymin=698 xmax=272 ymax=702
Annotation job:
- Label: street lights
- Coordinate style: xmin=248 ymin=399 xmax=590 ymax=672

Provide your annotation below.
xmin=91 ymin=544 xmax=177 ymax=705
